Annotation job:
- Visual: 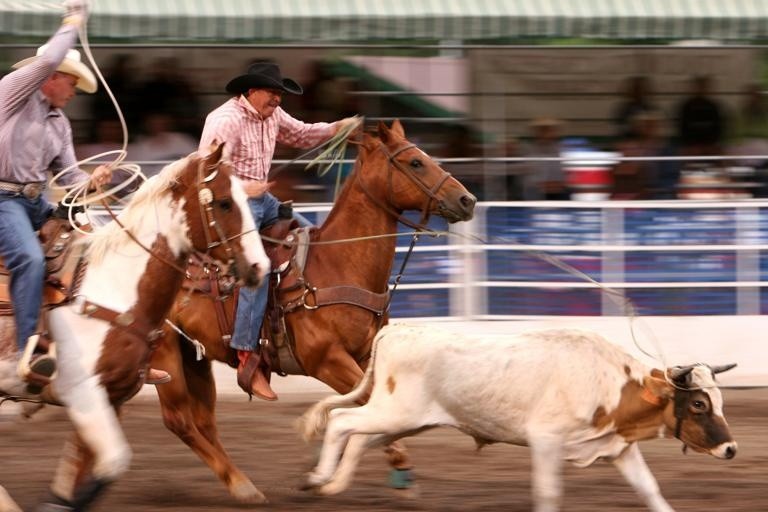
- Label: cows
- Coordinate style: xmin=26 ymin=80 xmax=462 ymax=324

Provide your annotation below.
xmin=296 ymin=316 xmax=739 ymax=511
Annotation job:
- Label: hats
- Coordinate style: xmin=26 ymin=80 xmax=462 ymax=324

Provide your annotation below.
xmin=225 ymin=62 xmax=303 ymax=95
xmin=11 ymin=44 xmax=98 ymax=94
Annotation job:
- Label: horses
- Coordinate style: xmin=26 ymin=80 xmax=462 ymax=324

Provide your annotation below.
xmin=148 ymin=118 xmax=480 ymax=507
xmin=0 ymin=136 xmax=278 ymax=512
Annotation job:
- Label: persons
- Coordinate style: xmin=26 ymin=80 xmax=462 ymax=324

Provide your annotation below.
xmin=0 ymin=0 xmax=115 ymax=396
xmin=193 ymin=60 xmax=365 ymax=401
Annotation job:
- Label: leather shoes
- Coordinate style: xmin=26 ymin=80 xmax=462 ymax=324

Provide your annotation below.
xmin=24 ymin=348 xmax=56 ymax=394
xmin=145 ymin=366 xmax=171 ymax=384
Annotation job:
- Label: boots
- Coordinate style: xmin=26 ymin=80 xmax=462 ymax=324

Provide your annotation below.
xmin=237 ymin=350 xmax=278 ymax=400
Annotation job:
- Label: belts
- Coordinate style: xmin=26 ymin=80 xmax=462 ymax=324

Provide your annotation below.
xmin=0 ymin=181 xmax=46 ymax=200
